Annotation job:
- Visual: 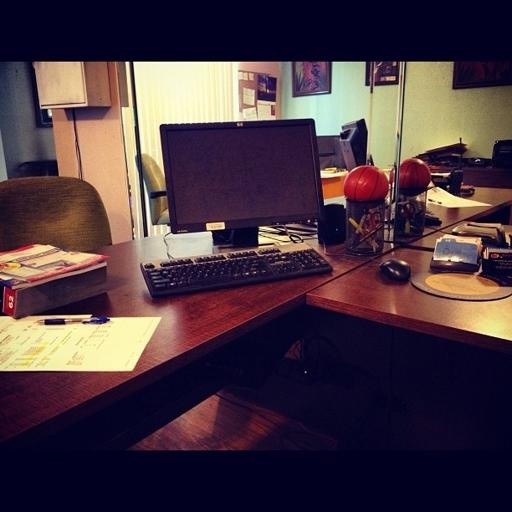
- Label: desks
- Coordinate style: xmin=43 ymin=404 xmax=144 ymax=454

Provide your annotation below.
xmin=406 ymin=219 xmax=512 ymax=260
xmin=0 ymin=181 xmax=511 ymax=511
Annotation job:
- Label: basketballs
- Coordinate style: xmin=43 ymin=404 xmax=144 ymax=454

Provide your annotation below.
xmin=345 ymin=165 xmax=388 ymax=201
xmin=399 ymin=159 xmax=431 ymax=193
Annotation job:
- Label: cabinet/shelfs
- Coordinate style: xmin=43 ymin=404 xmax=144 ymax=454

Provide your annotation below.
xmin=427 ymin=167 xmax=512 ymax=189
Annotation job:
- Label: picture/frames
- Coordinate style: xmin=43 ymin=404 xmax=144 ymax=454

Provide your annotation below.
xmin=452 ymin=61 xmax=511 ymax=90
xmin=365 ymin=62 xmax=398 ymax=87
xmin=289 ymin=62 xmax=331 ymax=97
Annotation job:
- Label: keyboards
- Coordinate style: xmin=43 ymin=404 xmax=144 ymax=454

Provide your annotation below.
xmin=139 ymin=241 xmax=336 ymax=300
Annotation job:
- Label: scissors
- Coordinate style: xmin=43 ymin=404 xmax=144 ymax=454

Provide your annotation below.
xmin=398 ymin=202 xmax=418 ymax=227
xmin=365 ymin=212 xmax=382 ymax=242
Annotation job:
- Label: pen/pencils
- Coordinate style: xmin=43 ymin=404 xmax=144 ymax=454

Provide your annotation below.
xmin=415 ymin=210 xmax=424 ymax=217
xmin=34 ymin=314 xmax=110 ymax=325
xmin=349 ymin=208 xmax=384 ymax=250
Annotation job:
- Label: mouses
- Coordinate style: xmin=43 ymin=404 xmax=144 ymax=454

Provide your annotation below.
xmin=380 ymin=258 xmax=413 ymax=282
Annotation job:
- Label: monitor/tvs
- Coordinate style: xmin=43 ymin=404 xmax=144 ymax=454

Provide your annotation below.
xmin=158 ymin=117 xmax=325 ymax=249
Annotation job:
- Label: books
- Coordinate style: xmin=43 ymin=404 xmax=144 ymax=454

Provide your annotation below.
xmin=433 ymin=218 xmax=512 ymax=274
xmin=0 ymin=242 xmax=112 ymax=319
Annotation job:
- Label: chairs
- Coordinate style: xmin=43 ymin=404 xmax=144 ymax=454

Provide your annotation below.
xmin=1 ymin=174 xmax=115 ymax=258
xmin=137 ymin=150 xmax=172 ymax=225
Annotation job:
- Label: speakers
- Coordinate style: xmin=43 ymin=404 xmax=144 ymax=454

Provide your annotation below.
xmin=317 ymin=203 xmax=346 ymax=245
